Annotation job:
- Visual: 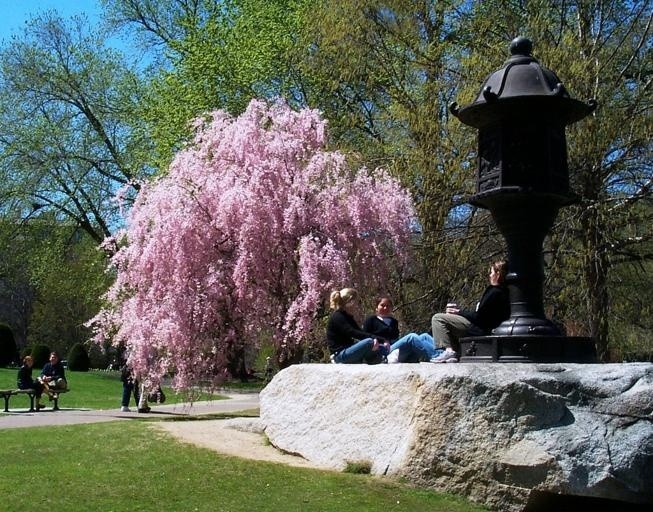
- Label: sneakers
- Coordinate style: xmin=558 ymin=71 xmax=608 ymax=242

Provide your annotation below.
xmin=365 ymin=352 xmax=387 ymax=364
xmin=138 ymin=407 xmax=151 ymax=413
xmin=429 ymin=350 xmax=459 ymax=362
xmin=121 ymin=406 xmax=130 ymax=412
xmin=36 ymin=404 xmax=45 ymax=408
xmin=49 ymin=394 xmax=58 ymax=401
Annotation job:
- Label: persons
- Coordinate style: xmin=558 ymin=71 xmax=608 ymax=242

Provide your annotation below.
xmin=325 ymin=287 xmax=391 ymax=364
xmin=17 ymin=356 xmax=59 ymax=409
xmin=36 ymin=352 xmax=67 ymax=389
xmin=119 ymin=366 xmax=151 ymax=412
xmin=430 ymin=259 xmax=510 ymax=363
xmin=361 ymin=296 xmax=457 ymax=363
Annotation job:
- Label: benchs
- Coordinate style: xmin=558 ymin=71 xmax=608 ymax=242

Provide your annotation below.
xmin=0 ymin=389 xmax=70 ymax=412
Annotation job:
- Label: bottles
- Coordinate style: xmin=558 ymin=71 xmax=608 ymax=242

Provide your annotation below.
xmin=447 ymin=303 xmax=458 ymax=309
